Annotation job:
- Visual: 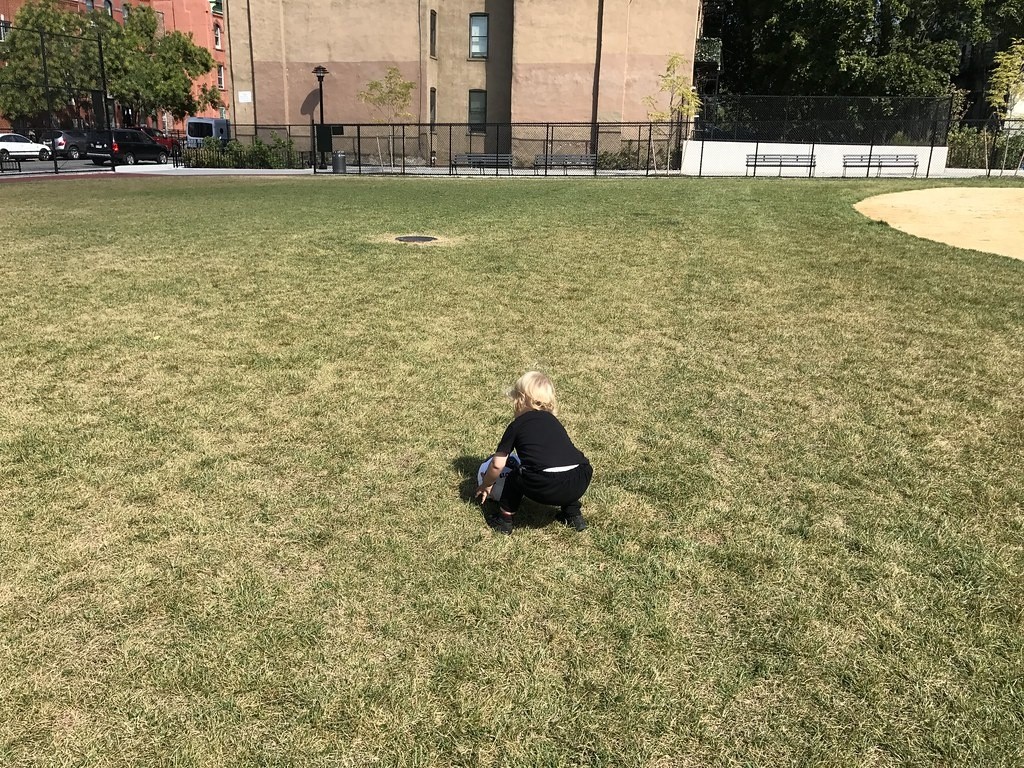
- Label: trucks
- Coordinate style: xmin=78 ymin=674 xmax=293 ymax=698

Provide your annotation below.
xmin=186 ymin=117 xmax=232 ymax=148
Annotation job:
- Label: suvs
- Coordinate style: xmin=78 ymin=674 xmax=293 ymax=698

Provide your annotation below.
xmin=125 ymin=128 xmax=181 ymax=157
xmin=0 ymin=132 xmax=52 ymax=162
xmin=39 ymin=129 xmax=87 ymax=160
xmin=85 ymin=129 xmax=169 ymax=165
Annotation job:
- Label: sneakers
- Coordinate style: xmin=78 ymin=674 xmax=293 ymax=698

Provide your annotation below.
xmin=486 ymin=510 xmax=513 ymax=535
xmin=556 ymin=512 xmax=585 ymax=531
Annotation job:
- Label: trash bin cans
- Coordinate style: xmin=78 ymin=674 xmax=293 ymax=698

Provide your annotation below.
xmin=331 ymin=149 xmax=346 ymax=174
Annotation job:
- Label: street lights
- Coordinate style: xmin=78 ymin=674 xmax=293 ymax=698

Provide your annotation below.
xmin=312 ymin=65 xmax=329 ymax=170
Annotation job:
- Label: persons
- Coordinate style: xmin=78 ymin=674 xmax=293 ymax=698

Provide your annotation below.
xmin=472 ymin=371 xmax=593 ymax=532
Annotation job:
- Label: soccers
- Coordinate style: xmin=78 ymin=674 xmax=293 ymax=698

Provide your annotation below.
xmin=476 ymin=452 xmax=528 ymax=504
